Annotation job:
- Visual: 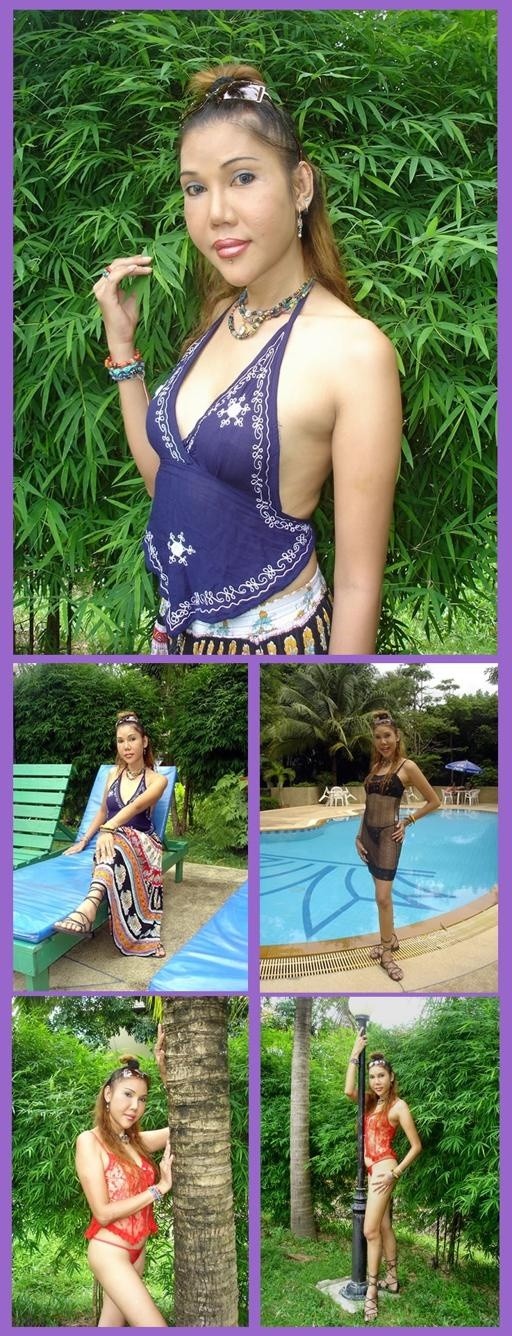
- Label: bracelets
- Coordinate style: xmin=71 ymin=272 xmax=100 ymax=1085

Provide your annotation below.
xmin=348 ymin=1057 xmax=360 ymax=1065
xmin=148 ymin=1185 xmax=164 ymax=1202
xmin=80 ymin=837 xmax=91 ymax=844
xmin=408 ymin=813 xmax=416 ymax=824
xmin=355 ymin=835 xmax=361 ymax=840
xmin=389 ymin=1168 xmax=402 ymax=1179
xmin=100 ymin=829 xmax=116 ymax=834
xmin=98 ymin=348 xmax=150 ymax=383
xmin=100 ymin=824 xmax=118 ymax=831
xmin=404 ymin=817 xmax=412 ymax=823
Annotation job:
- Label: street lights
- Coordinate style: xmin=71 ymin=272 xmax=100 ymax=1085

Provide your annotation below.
xmin=344 ymin=996 xmax=371 ymax=1300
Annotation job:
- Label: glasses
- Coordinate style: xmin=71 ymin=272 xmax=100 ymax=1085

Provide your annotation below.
xmin=174 ymin=77 xmax=278 ymax=125
xmin=115 ymin=714 xmax=139 ymax=727
xmin=111 ymin=1068 xmax=149 ymax=1084
xmin=368 ymin=1059 xmax=387 ymax=1068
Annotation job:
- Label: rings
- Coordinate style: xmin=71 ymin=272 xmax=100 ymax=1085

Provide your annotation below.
xmin=99 ymin=265 xmax=112 ymax=273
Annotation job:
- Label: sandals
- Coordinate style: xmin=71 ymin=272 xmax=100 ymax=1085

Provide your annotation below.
xmin=369 ymin=930 xmax=405 ymax=982
xmin=364 ymin=1274 xmax=378 ymax=1323
xmin=54 ymin=886 xmax=105 ymax=938
xmin=379 ymin=1258 xmax=399 ymax=1295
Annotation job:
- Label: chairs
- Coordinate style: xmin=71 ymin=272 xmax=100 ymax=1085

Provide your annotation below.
xmin=440 ymin=786 xmax=480 ymax=807
xmin=318 ymin=785 xmax=357 ymax=808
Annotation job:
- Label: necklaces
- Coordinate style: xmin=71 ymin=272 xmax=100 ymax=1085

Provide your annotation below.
xmin=226 ymin=273 xmax=316 ymax=345
xmin=124 ymin=764 xmax=147 ymax=780
xmin=126 ymin=767 xmax=143 ymax=776
xmin=376 ymin=1099 xmax=386 ymax=1106
xmin=380 ymin=757 xmax=393 ymax=768
xmin=114 ymin=1131 xmax=133 ymax=1145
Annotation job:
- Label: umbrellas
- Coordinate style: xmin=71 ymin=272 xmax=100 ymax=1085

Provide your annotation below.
xmin=445 ymin=759 xmax=482 ymax=787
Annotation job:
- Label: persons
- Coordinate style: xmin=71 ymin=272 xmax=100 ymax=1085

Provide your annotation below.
xmin=72 ymin=1056 xmax=176 ymax=1329
xmin=55 ymin=707 xmax=170 ymax=940
xmin=91 ymin=66 xmax=399 ymax=654
xmin=445 ymin=781 xmax=457 ymax=804
xmin=353 ymin=705 xmax=444 ymax=982
xmin=345 ymin=1026 xmax=423 ymax=1324
